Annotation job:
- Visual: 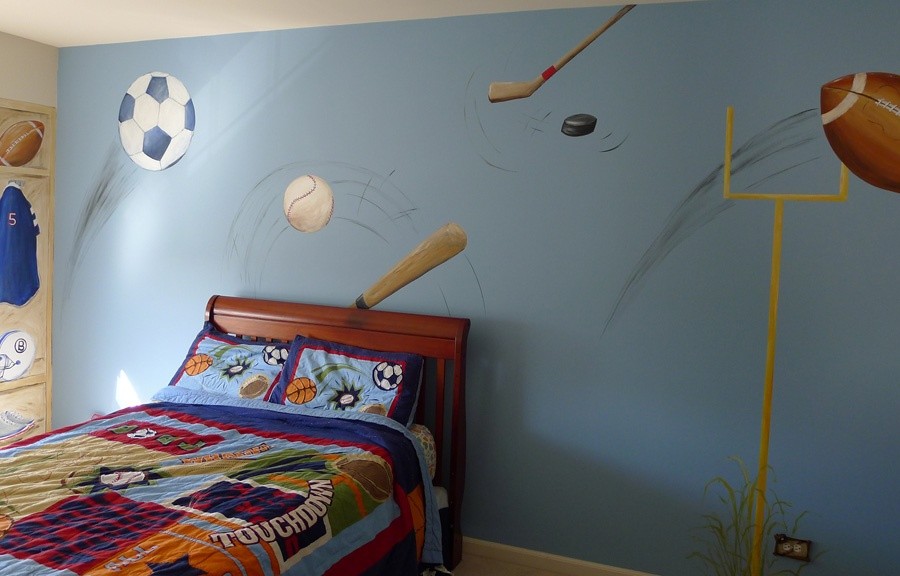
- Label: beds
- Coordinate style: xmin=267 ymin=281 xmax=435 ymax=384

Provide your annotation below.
xmin=0 ymin=295 xmax=471 ymax=576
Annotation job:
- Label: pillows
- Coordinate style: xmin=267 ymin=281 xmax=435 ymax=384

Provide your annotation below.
xmin=168 ymin=321 xmax=292 ymax=404
xmin=277 ymin=334 xmax=424 ymax=428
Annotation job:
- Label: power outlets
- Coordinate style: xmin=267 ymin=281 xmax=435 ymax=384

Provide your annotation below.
xmin=774 ymin=534 xmax=812 ymax=562
